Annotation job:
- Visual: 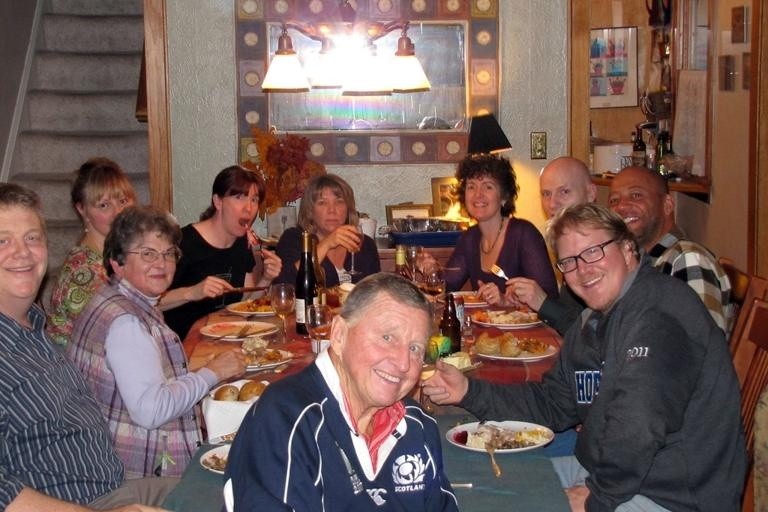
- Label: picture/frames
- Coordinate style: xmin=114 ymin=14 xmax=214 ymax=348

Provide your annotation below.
xmin=431 ymin=176 xmax=478 ymax=230
xmin=385 ymin=203 xmax=435 ymax=249
xmin=235 ymin=2 xmax=500 ymax=166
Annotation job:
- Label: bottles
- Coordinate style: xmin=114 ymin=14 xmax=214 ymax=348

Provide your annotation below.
xmin=293 ymin=231 xmax=318 ymax=334
xmin=633 ymin=126 xmax=674 ymax=177
xmin=441 ymin=294 xmax=462 ymax=357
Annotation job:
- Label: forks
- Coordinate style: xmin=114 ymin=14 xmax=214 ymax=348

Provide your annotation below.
xmin=483 ymin=431 xmax=504 ymax=478
xmin=213 ymin=325 xmax=250 ymax=340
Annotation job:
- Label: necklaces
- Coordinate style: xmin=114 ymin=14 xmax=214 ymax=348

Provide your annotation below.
xmin=476 ymin=217 xmax=506 ymax=254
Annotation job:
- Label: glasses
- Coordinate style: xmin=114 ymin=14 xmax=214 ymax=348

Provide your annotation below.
xmin=126 ymin=246 xmax=181 ymax=262
xmin=556 ymin=237 xmax=618 ymax=274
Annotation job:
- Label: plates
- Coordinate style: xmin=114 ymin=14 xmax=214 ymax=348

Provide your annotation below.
xmin=446 ymin=419 xmax=557 ymax=454
xmin=208 ymin=347 xmax=293 ymax=372
xmin=475 ymin=341 xmax=559 ymax=363
xmin=227 ymin=299 xmax=274 ymax=317
xmin=200 ymin=444 xmax=232 ymax=475
xmin=469 ymin=310 xmax=543 ymax=331
xmin=450 ymin=290 xmax=490 ymax=309
xmin=200 ymin=321 xmax=278 ymax=341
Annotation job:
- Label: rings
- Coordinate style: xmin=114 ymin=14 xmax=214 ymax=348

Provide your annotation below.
xmin=486 ymin=284 xmax=490 ymax=288
xmin=488 ymin=294 xmax=492 ymax=298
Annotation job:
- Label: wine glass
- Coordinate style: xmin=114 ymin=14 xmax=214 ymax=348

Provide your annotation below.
xmin=307 ymin=305 xmax=330 ymax=357
xmin=271 ymin=225 xmax=447 ymax=416
xmin=272 ymin=283 xmax=295 ymax=346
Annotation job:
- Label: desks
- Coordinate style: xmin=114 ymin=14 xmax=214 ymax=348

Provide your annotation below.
xmin=376 ymin=246 xmax=473 ymax=291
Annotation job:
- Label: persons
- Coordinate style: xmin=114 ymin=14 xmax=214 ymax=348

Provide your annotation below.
xmin=505 ymin=157 xmax=597 ymax=338
xmin=224 ymin=273 xmax=458 ymax=511
xmin=39 ymin=156 xmax=232 ymax=348
xmin=610 ymin=167 xmax=738 ymax=345
xmin=66 ymin=203 xmax=246 ymax=482
xmin=1 ymin=186 xmax=185 ymax=512
xmin=408 ymin=204 xmax=752 ymax=511
xmin=161 ymin=168 xmax=283 ymax=341
xmin=270 ymin=174 xmax=380 ymax=302
xmin=412 ymin=154 xmax=561 ymax=304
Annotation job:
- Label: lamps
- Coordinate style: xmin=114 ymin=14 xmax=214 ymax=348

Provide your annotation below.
xmin=260 ymin=19 xmax=432 ymax=98
xmin=467 ymin=113 xmax=513 ymax=158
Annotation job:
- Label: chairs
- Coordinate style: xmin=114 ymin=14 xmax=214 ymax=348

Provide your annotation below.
xmin=717 ymin=256 xmax=768 ymax=512
